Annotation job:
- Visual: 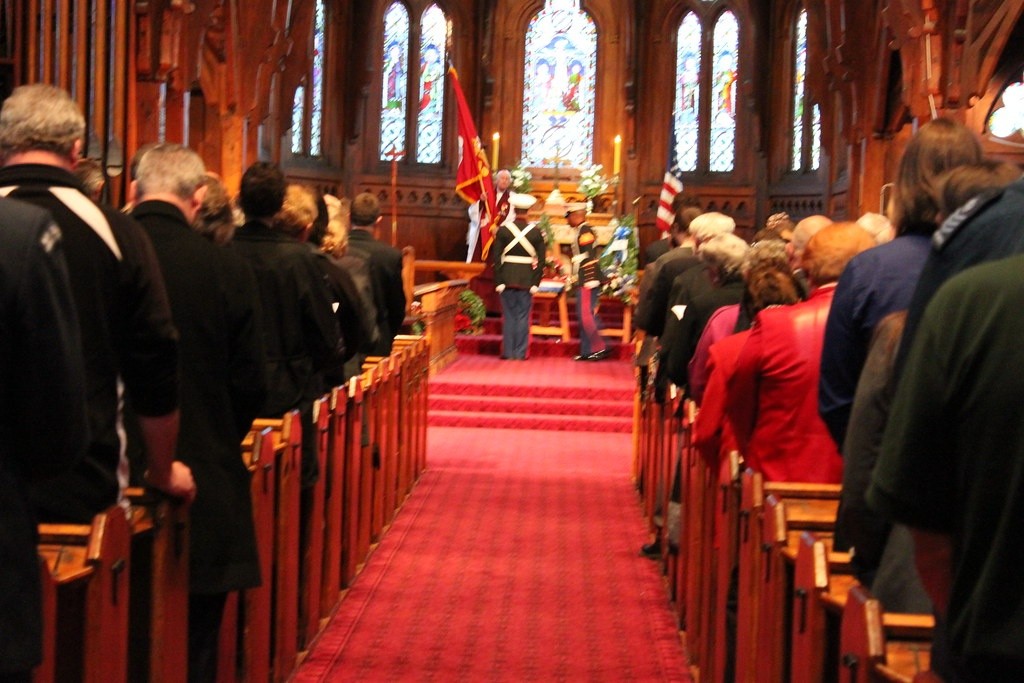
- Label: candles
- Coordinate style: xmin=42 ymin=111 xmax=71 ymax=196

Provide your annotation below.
xmin=492 ymin=133 xmax=500 ymax=172
xmin=615 ymin=134 xmax=621 ymax=175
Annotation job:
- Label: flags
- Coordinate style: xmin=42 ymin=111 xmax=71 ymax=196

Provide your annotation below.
xmin=447 ymin=65 xmax=508 ymax=261
xmin=654 ymin=114 xmax=684 ymax=235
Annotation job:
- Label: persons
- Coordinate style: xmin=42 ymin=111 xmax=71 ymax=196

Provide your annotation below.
xmin=468 ymin=169 xmax=518 ymax=263
xmin=639 ymin=116 xmax=1024 ymax=683
xmin=561 ymin=202 xmax=611 ymax=361
xmin=493 ymin=194 xmax=545 ymax=360
xmin=0 ymin=84 xmax=409 ymax=683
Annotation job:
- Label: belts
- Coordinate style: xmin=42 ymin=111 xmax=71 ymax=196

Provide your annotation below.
xmin=570 ymin=251 xmax=589 ymax=263
xmin=501 ymin=254 xmax=535 ymax=265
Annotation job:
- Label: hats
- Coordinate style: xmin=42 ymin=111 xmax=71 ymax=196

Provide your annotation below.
xmin=508 ymin=193 xmax=537 ymax=211
xmin=563 ymin=201 xmax=587 ymax=219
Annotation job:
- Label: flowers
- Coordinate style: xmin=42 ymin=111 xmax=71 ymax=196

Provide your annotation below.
xmin=598 ymin=214 xmax=640 ymax=307
xmin=539 ymin=212 xmax=569 ymax=283
xmin=509 ymin=162 xmax=533 ymax=195
xmin=576 ymin=164 xmax=609 ymax=200
xmin=454 ymin=290 xmax=487 ymax=336
xmin=410 ymin=301 xmax=423 ymax=335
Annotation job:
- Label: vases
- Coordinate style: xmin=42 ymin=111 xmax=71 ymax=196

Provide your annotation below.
xmin=585 ymin=199 xmax=594 ymax=216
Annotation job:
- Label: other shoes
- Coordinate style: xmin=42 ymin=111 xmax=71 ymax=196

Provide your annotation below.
xmin=642 ymin=541 xmax=666 ymax=558
xmin=499 ymin=354 xmax=513 ymax=360
xmin=589 ymin=349 xmax=610 ymax=360
xmin=574 ymin=355 xmax=591 ymax=361
